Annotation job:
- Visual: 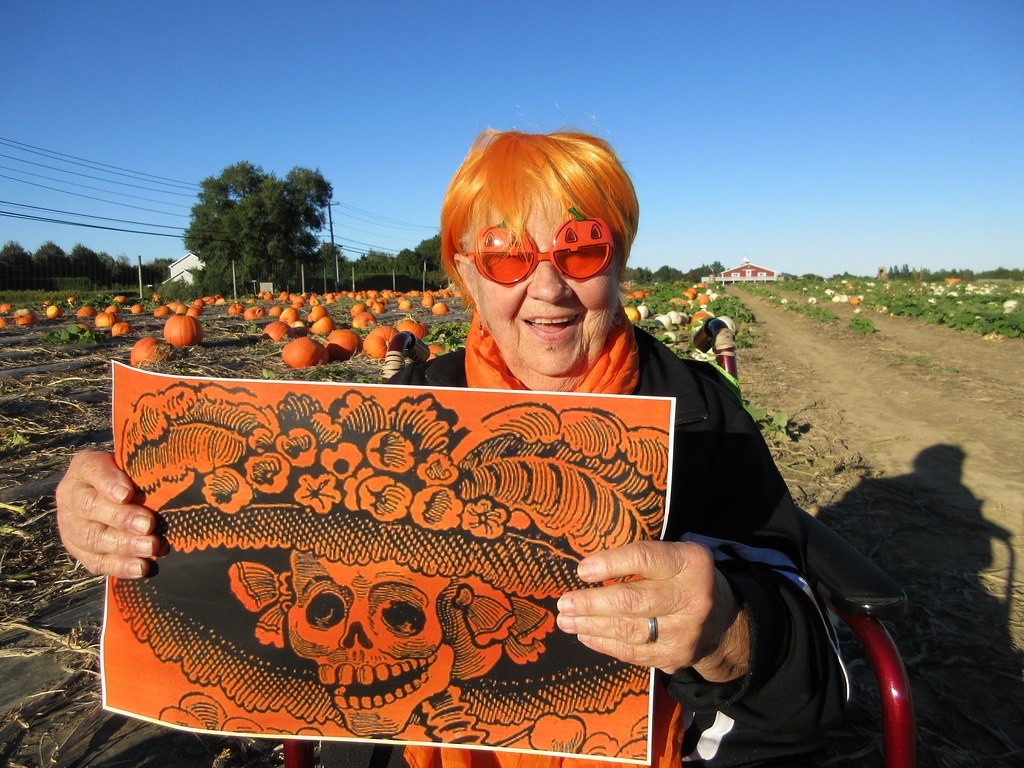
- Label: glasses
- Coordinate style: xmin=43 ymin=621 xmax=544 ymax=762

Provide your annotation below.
xmin=465 ymin=207 xmax=619 ymax=286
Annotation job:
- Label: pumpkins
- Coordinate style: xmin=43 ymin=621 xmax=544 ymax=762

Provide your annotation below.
xmin=0 ymin=290 xmax=461 ymax=370
xmin=618 ymin=282 xmax=737 ymax=346
xmin=778 ymin=281 xmax=1024 ymax=316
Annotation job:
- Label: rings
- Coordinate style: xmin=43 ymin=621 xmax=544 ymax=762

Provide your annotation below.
xmin=649 ymin=618 xmax=658 ymax=643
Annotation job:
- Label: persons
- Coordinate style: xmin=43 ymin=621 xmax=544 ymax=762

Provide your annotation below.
xmin=56 ymin=131 xmax=837 ymax=768
